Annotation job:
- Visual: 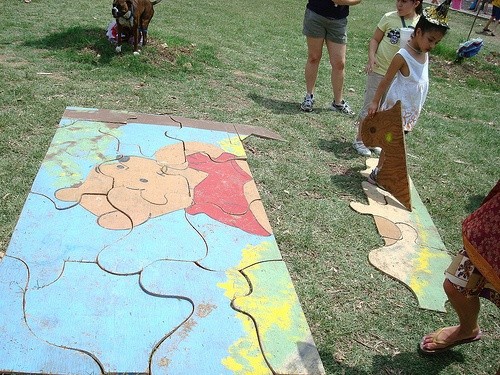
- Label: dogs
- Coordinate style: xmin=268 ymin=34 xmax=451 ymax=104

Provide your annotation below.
xmin=111 ymin=0 xmax=162 ymax=54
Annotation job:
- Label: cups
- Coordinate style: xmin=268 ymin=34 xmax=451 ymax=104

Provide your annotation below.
xmin=400 ymin=28 xmax=414 ymax=48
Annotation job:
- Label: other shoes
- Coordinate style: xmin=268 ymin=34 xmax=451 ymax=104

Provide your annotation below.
xmin=483 ymin=28 xmax=491 ymax=32
xmin=492 ymin=32 xmax=496 ymax=36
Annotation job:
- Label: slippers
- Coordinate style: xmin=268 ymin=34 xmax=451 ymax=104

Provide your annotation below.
xmin=419 ymin=327 xmax=482 ymax=353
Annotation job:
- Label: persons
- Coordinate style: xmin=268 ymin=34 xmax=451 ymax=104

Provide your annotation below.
xmin=353 ymin=0 xmax=424 ymax=156
xmin=366 ymin=0 xmax=453 ymax=191
xmin=300 ymin=0 xmax=362 ymax=117
xmin=432 ymin=0 xmax=500 ymax=35
xmin=420 ymin=179 xmax=500 ymax=354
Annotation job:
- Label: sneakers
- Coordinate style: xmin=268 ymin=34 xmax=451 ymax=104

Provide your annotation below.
xmin=352 ymin=138 xmax=371 ymax=156
xmin=300 ymin=94 xmax=314 ymax=111
xmin=368 ymin=170 xmax=387 ymax=191
xmin=369 ymin=146 xmax=382 ymax=154
xmin=330 ymin=99 xmax=354 ymax=115
